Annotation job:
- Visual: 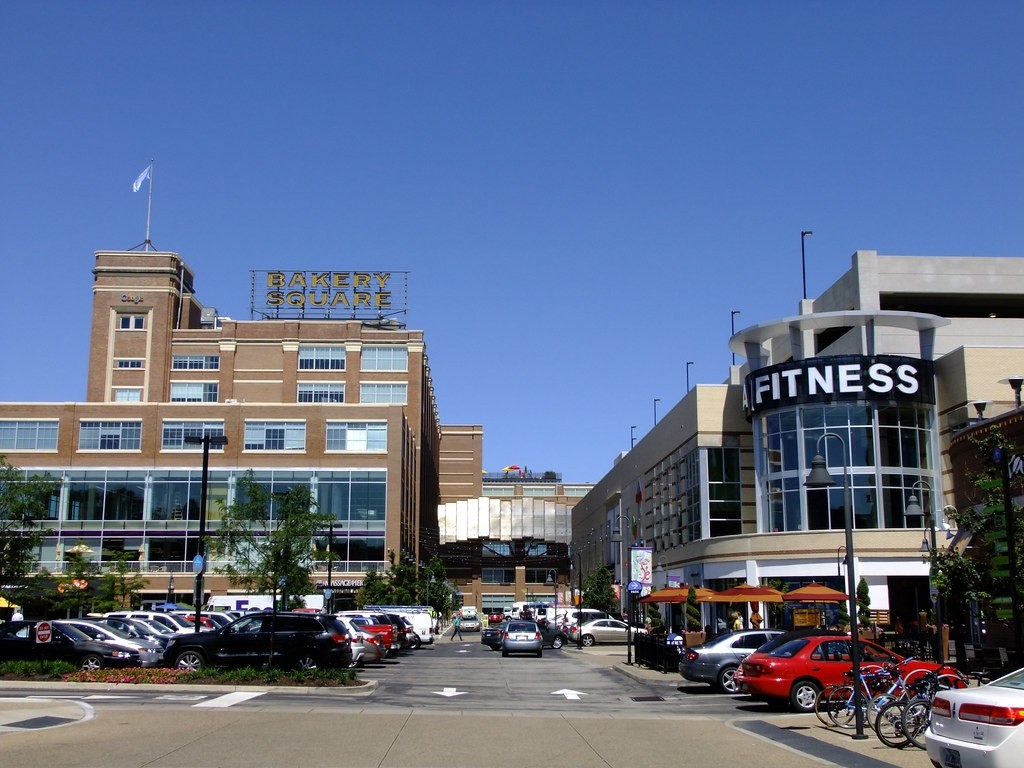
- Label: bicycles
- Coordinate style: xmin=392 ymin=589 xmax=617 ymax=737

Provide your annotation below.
xmin=814 ymin=655 xmax=992 ymax=753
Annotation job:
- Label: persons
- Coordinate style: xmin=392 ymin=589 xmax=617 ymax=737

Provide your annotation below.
xmin=666 ymin=627 xmax=685 ymax=656
xmin=451 ymin=612 xmax=456 ymax=621
xmin=457 ymin=609 xmax=462 ymax=618
xmin=466 ymin=610 xmax=472 ymax=617
xmin=731 ymin=611 xmax=743 ymax=630
xmin=450 ymin=615 xmax=465 ymax=641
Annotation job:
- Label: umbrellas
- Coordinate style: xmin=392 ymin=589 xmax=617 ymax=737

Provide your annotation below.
xmin=65 ymin=543 xmax=95 ymax=553
xmin=0 ymin=596 xmax=20 ymax=608
xmin=695 ymin=583 xmax=796 ymax=602
xmin=639 ymin=585 xmax=718 ymax=631
xmin=782 ymin=583 xmax=862 ymax=629
xmin=151 ymin=601 xmax=196 ymax=611
xmin=502 ymin=465 xmax=520 ymax=471
xmin=482 ymin=469 xmax=487 ymax=474
xmin=750 ymin=601 xmax=765 ymax=629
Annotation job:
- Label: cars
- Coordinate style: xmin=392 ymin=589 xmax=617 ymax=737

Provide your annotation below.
xmin=732 ymin=628 xmax=969 ymax=714
xmin=46 ymin=594 xmax=438 ymax=670
xmin=458 ymin=606 xmax=481 ymax=632
xmin=503 ymin=601 xmax=617 ymax=637
xmin=0 ymin=620 xmax=143 ymax=675
xmin=500 ymin=621 xmax=544 ymax=658
xmin=923 ymin=665 xmax=1024 ymax=768
xmin=488 ymin=612 xmax=502 ymax=625
xmin=676 ymin=629 xmax=792 ymax=693
xmin=566 ymin=618 xmax=647 ymax=646
xmin=481 ymin=619 xmax=569 ymax=652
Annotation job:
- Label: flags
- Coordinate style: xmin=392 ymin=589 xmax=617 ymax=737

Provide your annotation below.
xmin=133 ymin=163 xmax=150 ymax=192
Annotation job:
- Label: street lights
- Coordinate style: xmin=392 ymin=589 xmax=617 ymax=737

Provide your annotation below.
xmin=731 ymin=310 xmax=740 ymax=366
xmin=900 ymin=479 xmax=944 ymax=666
xmin=653 ymin=398 xmax=661 ymax=425
xmin=569 ymin=551 xmax=584 ymax=650
xmin=611 ymin=515 xmax=633 ymax=667
xmin=630 ymin=425 xmax=637 ymax=449
xmin=183 ymin=434 xmax=229 ymax=634
xmin=836 ymin=545 xmax=849 ymax=592
xmin=320 ymin=522 xmax=343 ymax=588
xmin=544 ymin=568 xmax=558 ymax=632
xmin=686 ymin=361 xmax=694 ymax=392
xmin=800 ymin=229 xmax=813 ymax=298
xmin=803 ymin=432 xmax=868 ymax=740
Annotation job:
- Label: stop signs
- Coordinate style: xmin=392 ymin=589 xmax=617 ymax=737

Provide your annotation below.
xmin=37 ymin=622 xmax=51 ymax=641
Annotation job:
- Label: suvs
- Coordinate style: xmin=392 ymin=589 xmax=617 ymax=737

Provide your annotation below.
xmin=162 ymin=612 xmax=355 ymax=674
